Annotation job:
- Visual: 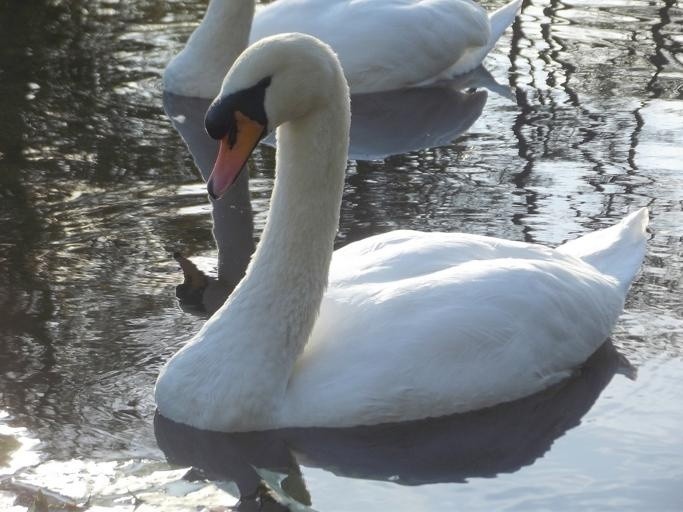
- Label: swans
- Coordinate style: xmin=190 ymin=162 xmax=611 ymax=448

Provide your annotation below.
xmin=162 ymin=1 xmax=523 ymax=99
xmin=159 ymin=64 xmax=516 ymax=321
xmin=153 ymin=32 xmax=649 ymax=432
